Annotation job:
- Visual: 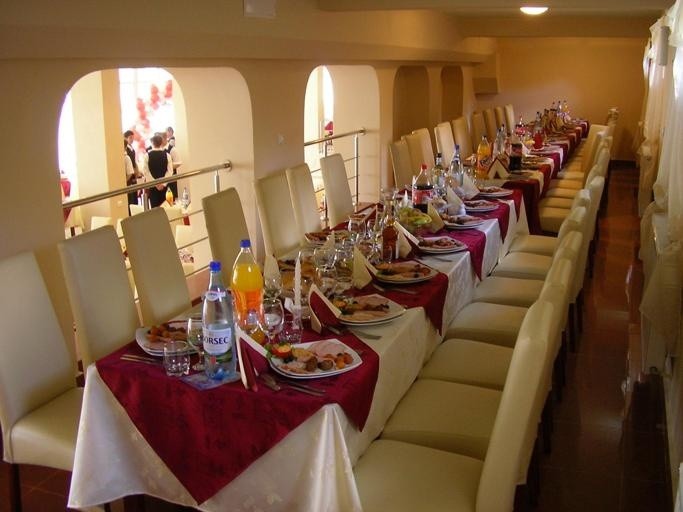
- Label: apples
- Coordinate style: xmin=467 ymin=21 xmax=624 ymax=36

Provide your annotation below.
xmin=411 ymin=209 xmax=421 ymax=217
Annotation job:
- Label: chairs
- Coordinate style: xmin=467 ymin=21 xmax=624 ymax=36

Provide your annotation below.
xmin=1 ymin=104 xmax=618 ymax=511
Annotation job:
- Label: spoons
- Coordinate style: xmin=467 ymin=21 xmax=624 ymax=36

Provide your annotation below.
xmin=259 ymin=372 xmax=325 ymax=397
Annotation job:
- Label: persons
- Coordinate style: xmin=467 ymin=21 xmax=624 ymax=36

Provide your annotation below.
xmin=123 ymin=124 xmax=176 ymax=218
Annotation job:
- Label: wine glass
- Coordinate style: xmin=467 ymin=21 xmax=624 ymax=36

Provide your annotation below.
xmin=186 ymin=313 xmax=205 ymax=371
xmin=258 ymin=296 xmax=285 ymax=345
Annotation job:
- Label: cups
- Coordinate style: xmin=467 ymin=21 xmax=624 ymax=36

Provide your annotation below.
xmin=263 ymin=186 xmax=399 ymax=315
xmin=281 ymin=312 xmax=304 ymax=344
xmin=161 ymin=340 xmax=189 ymax=377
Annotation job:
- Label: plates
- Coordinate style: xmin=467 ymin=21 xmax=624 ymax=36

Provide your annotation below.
xmin=332 ymin=293 xmax=407 ymax=326
xmin=372 ymin=260 xmax=438 ymax=285
xmin=414 ymin=236 xmax=469 ymax=255
xmin=268 ymin=338 xmax=363 ymax=380
xmin=442 ymin=215 xmax=485 ymax=229
xmin=134 ymin=323 xmax=196 ymax=357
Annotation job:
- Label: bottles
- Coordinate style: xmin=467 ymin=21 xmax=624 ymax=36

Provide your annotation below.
xmin=165 ymin=186 xmax=173 ymax=207
xmin=228 ymin=237 xmax=264 ymax=345
xmin=411 ymin=99 xmax=570 ymax=213
xmin=200 ymin=261 xmax=233 ymax=382
xmin=182 ymin=187 xmax=189 ymax=202
